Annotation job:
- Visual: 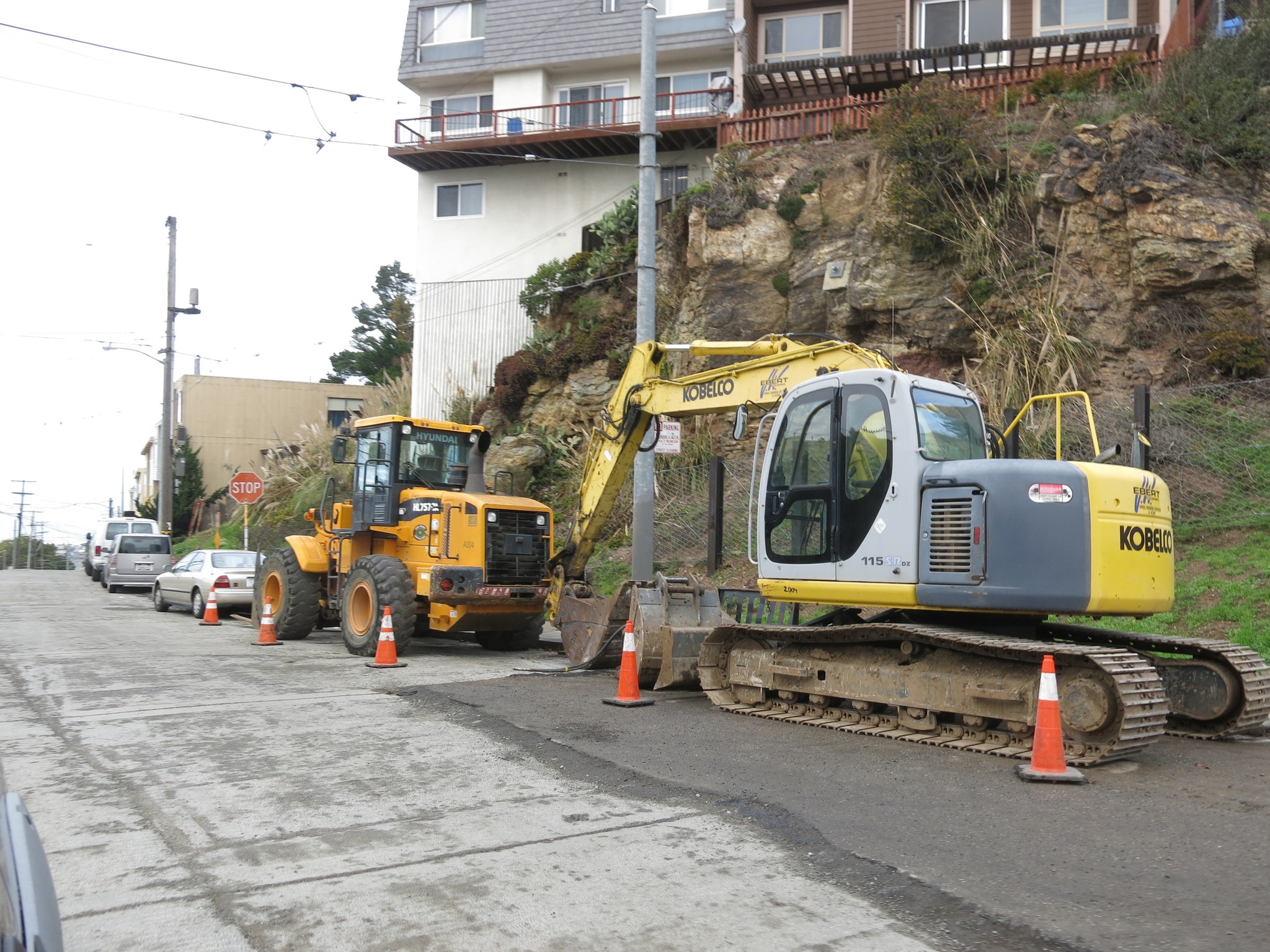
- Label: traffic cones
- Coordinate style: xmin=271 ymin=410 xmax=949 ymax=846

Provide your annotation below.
xmin=199 ymin=586 xmax=223 ymax=626
xmin=365 ymin=606 xmax=408 ymax=667
xmin=599 ymin=620 xmax=656 ymax=708
xmin=1018 ymin=653 xmax=1086 ymax=783
xmin=250 ymin=595 xmax=284 ymax=646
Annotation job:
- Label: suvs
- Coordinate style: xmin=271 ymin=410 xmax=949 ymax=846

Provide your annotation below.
xmin=101 ymin=533 xmax=175 ymax=594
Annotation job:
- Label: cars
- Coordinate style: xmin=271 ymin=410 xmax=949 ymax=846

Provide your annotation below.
xmin=151 ymin=549 xmax=267 ymax=618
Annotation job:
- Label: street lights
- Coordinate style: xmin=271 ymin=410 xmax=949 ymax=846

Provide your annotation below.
xmin=102 ymin=345 xmax=173 ymax=538
xmin=73 ymin=497 xmax=113 ymax=518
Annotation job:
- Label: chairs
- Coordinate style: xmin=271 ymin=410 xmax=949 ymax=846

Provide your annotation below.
xmin=150 ymin=544 xmax=161 ymax=554
xmin=411 ymin=455 xmax=442 ymax=483
xmin=124 ymin=544 xmax=135 ymax=553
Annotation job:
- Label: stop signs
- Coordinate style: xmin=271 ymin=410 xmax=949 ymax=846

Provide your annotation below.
xmin=229 ymin=472 xmax=263 ymax=504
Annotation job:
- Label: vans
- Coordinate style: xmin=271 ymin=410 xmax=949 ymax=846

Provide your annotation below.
xmin=82 ymin=516 xmax=163 ymax=581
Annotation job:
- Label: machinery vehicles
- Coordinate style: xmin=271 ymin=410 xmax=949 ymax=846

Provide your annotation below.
xmin=255 ymin=413 xmax=555 ymax=659
xmin=537 ymin=332 xmax=1269 ymax=772
